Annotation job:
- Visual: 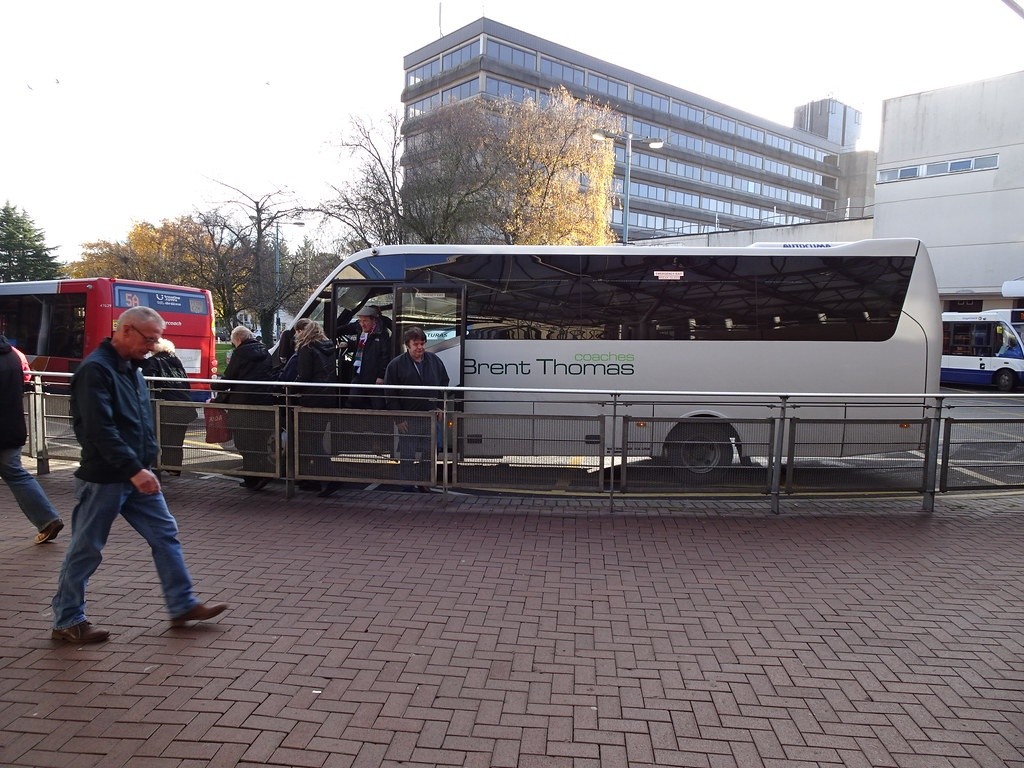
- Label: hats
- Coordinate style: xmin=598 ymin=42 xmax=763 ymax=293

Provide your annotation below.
xmin=357 ymin=307 xmax=382 ymax=319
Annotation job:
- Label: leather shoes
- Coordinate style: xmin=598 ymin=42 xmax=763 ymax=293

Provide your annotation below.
xmin=169 ymin=602 xmax=227 ymax=627
xmin=52 ymin=620 xmax=111 ymax=642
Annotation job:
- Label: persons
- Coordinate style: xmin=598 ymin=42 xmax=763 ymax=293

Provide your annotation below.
xmin=11 ymin=346 xmax=32 ymax=384
xmin=51 ymin=306 xmax=227 ymax=645
xmin=0 ymin=336 xmax=64 ymax=544
xmin=142 ymin=337 xmax=191 ymax=476
xmin=275 ymin=317 xmax=341 ymax=498
xmin=384 ymin=327 xmax=450 ymax=493
xmin=210 ymin=325 xmax=276 ymax=490
xmin=337 ymin=305 xmax=393 ymax=451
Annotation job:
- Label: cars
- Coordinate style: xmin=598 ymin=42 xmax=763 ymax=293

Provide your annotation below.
xmin=216 ymin=326 xmax=277 ymax=343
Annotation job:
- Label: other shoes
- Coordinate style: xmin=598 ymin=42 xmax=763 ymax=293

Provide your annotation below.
xmin=238 ymin=481 xmax=248 ymax=486
xmin=419 ymin=483 xmax=434 ymax=493
xmin=318 ymin=482 xmax=343 ymax=498
xmin=253 ymin=476 xmax=273 ymax=492
xmin=162 ymin=468 xmax=181 ymax=476
xmin=404 ymin=483 xmax=419 ymax=492
xmin=32 ymin=519 xmax=64 ymax=544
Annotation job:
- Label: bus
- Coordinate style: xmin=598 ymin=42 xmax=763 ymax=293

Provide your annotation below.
xmin=940 ymin=309 xmax=1024 ymax=393
xmin=0 ymin=277 xmax=218 ymax=408
xmin=268 ymin=236 xmax=944 ymax=487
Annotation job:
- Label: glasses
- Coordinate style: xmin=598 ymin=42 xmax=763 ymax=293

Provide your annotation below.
xmin=126 ymin=323 xmax=160 ymax=346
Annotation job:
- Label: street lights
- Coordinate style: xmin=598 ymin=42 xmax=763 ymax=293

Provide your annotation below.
xmin=275 ymin=222 xmax=307 ymax=342
xmin=592 ymin=127 xmax=664 ymax=246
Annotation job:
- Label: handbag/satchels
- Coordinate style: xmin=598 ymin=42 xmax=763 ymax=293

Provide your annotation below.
xmin=203 ymin=398 xmax=232 ymax=443
